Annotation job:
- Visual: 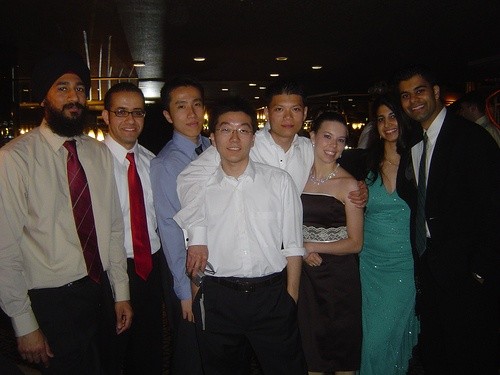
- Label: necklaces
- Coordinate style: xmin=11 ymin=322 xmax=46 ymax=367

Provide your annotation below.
xmin=308 ymin=164 xmax=341 ymax=186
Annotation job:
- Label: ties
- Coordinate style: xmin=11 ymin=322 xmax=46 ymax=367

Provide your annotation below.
xmin=125 ymin=152 xmax=153 ymax=281
xmin=62 ymin=139 xmax=105 ymax=287
xmin=414 ymin=132 xmax=429 ymax=258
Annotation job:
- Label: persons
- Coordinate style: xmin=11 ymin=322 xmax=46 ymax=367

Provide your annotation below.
xmin=101 ymin=83 xmax=174 ymax=375
xmin=300 ymin=112 xmax=364 ymax=374
xmin=172 ymin=79 xmax=369 ymax=279
xmin=0 ymin=47 xmax=133 ymax=375
xmin=358 ymin=90 xmax=422 ymax=375
xmin=171 ymin=97 xmax=309 ymax=375
xmin=396 ymin=63 xmax=500 ymax=375
xmin=150 ymin=79 xmax=216 ymax=375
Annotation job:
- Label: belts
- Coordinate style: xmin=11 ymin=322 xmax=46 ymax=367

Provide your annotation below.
xmin=28 ymin=275 xmax=90 ymax=296
xmin=209 ymin=271 xmax=284 ymax=292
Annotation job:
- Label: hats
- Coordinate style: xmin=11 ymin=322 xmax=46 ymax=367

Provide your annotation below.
xmin=23 ymin=47 xmax=91 ymax=105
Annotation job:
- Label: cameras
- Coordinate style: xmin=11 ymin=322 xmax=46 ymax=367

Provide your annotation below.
xmin=185 ymin=268 xmax=206 ymax=287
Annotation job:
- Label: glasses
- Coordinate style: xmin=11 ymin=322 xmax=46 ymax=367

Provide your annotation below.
xmin=215 ymin=128 xmax=253 ymax=136
xmin=107 ymin=109 xmax=146 ymax=119
